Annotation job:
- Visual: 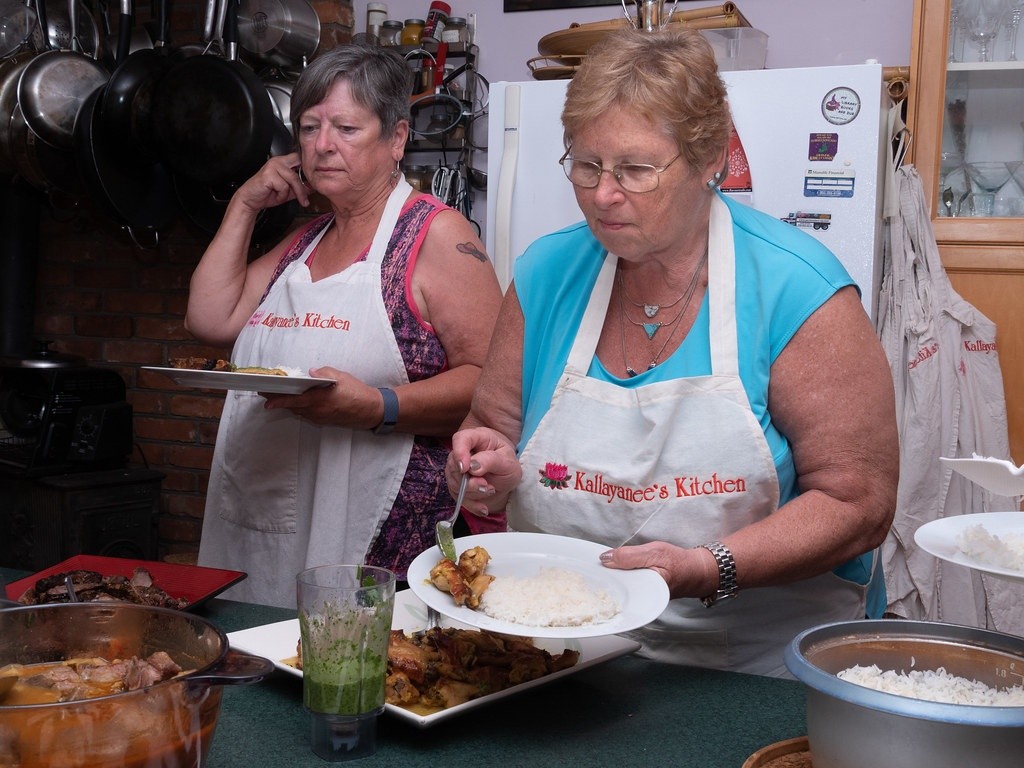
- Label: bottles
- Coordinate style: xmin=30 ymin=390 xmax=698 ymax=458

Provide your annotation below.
xmin=381 ymin=21 xmax=403 ymax=47
xmin=410 ymin=58 xmax=454 ymax=94
xmin=440 ymin=17 xmax=469 ymax=43
xmin=400 ymin=19 xmax=425 ymax=46
xmin=427 ymin=114 xmax=450 ymax=140
xmin=419 ymin=164 xmax=440 ymax=195
xmin=420 ymin=1 xmax=451 ymax=43
xmin=365 ymin=2 xmax=387 ymax=43
xmin=404 ymin=165 xmax=425 ymax=192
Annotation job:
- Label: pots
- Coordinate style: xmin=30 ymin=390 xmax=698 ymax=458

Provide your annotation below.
xmin=0 ymin=601 xmax=275 ymax=768
xmin=782 ymin=618 xmax=1024 ymax=767
xmin=1 ymin=0 xmax=321 ymax=252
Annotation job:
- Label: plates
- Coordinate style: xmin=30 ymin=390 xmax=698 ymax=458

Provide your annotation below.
xmin=226 ymin=587 xmax=642 ymax=725
xmin=139 ymin=365 xmax=337 ymax=395
xmin=913 ymin=511 xmax=1024 ymax=578
xmin=406 ymin=532 xmax=670 ymax=639
xmin=0 ymin=553 xmax=248 ymax=613
xmin=939 ymin=456 xmax=1024 ymax=496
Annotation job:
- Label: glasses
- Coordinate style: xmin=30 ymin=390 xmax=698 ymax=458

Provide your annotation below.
xmin=558 ymin=143 xmax=683 ymax=194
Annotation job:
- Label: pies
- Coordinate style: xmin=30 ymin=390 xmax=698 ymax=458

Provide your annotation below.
xmin=235 ymin=367 xmax=287 ymax=376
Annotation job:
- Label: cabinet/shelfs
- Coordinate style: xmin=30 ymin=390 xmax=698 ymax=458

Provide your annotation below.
xmin=903 ymin=0 xmax=1024 ymax=511
xmin=379 ymin=36 xmax=484 ymax=208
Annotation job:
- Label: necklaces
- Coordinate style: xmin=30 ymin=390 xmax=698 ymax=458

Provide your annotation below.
xmin=616 ymin=243 xmax=708 ymax=378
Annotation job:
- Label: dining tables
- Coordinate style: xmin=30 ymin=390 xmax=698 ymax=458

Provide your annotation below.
xmin=0 ymin=596 xmax=810 ymax=768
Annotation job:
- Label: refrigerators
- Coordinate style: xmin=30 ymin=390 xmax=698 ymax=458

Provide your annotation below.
xmin=485 ymin=59 xmax=894 ymax=336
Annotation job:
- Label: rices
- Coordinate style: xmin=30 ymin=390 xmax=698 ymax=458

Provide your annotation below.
xmin=972 ymin=452 xmax=1020 ymax=470
xmin=478 ymin=564 xmax=620 ymax=626
xmin=268 ymin=365 xmax=309 ymax=377
xmin=954 ymin=523 xmax=1023 ymax=570
xmin=833 ymin=657 xmax=1024 ymax=706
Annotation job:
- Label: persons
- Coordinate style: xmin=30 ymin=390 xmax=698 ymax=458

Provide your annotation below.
xmin=184 ymin=46 xmax=502 ymax=606
xmin=447 ymin=25 xmax=898 ymax=681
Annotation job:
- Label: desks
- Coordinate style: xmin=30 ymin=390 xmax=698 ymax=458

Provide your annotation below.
xmin=0 ymin=463 xmax=168 ymax=574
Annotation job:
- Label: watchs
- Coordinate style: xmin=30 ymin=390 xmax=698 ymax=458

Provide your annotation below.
xmin=694 ymin=540 xmax=738 ymax=609
xmin=365 ymin=387 xmax=400 ymax=436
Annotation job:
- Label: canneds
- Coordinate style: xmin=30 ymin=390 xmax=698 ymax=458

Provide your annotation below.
xmin=366 ymin=1 xmax=470 ymax=196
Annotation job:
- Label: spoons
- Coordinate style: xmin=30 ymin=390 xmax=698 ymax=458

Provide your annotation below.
xmin=435 ymin=473 xmax=467 ymax=561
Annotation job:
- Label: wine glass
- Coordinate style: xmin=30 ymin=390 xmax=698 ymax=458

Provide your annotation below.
xmin=949 ymin=1 xmax=1024 ymax=63
xmin=935 ymin=152 xmax=1024 ymax=216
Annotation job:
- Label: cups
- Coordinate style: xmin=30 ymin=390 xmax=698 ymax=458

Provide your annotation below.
xmin=295 ymin=563 xmax=396 ymax=761
